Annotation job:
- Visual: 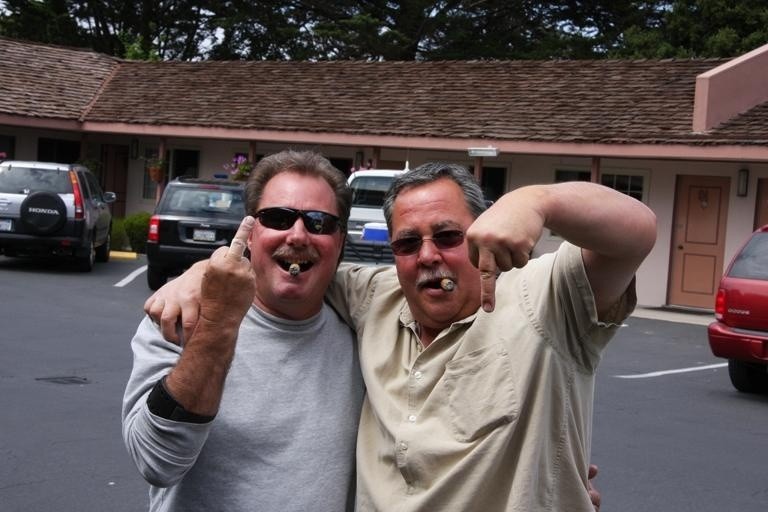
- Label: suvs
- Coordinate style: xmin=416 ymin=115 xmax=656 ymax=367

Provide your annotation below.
xmin=335 ymin=160 xmax=432 ymax=269
xmin=144 ymin=173 xmax=248 ymax=290
xmin=707 ymin=221 xmax=767 ymax=398
xmin=1 ymin=157 xmax=117 ymax=270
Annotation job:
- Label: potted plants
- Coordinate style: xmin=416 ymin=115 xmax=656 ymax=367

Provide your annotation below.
xmin=141 ymin=154 xmax=166 ymax=184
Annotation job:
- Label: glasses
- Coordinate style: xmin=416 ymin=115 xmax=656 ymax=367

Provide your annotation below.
xmin=390 ymin=229 xmax=465 ymax=257
xmin=255 ymin=207 xmax=342 ymax=234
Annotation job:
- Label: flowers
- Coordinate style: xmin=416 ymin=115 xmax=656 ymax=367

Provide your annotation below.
xmin=223 ymin=156 xmax=252 ymax=181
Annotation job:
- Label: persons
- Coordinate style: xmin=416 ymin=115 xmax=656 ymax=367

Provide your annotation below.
xmin=140 ymin=156 xmax=663 ymax=511
xmin=115 ymin=147 xmax=607 ymax=511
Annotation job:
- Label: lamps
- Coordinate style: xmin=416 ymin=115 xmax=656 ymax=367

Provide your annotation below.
xmin=467 ymin=146 xmax=501 ymax=158
xmin=737 ymin=169 xmax=748 ymax=197
